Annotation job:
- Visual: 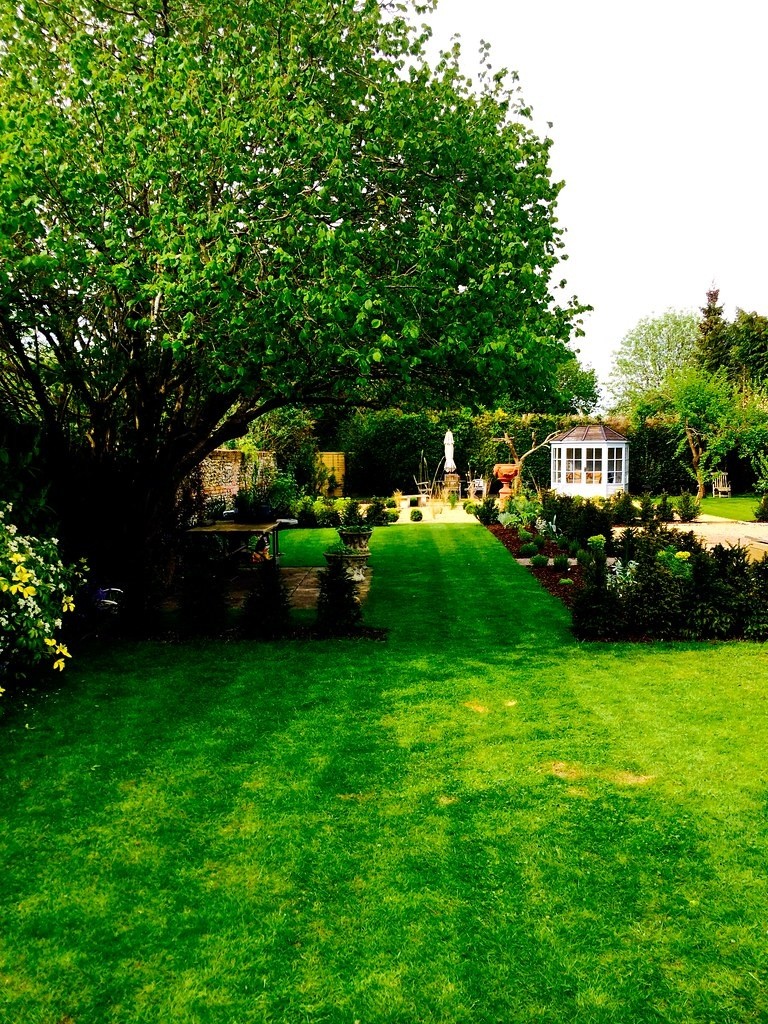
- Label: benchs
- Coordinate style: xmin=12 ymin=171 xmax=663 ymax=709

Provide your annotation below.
xmin=400 ymin=495 xmax=430 ymax=507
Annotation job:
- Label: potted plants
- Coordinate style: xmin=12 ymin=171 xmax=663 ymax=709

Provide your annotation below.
xmin=322 ymin=539 xmax=372 ymax=581
xmin=333 ymin=500 xmax=384 ymax=551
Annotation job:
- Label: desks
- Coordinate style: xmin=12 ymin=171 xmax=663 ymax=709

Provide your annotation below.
xmin=186 ymin=523 xmax=284 ymax=571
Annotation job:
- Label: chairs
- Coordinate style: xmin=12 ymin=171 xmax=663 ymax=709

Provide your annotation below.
xmin=710 ymin=472 xmax=731 ymax=499
